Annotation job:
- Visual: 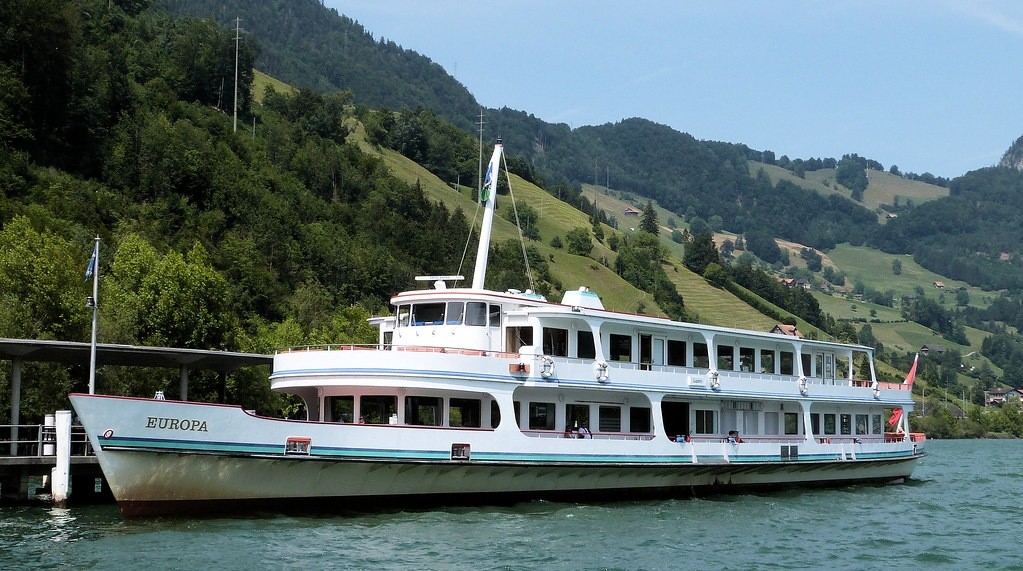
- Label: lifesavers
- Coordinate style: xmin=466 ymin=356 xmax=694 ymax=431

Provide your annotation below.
xmin=798 ymin=375 xmax=809 ymax=395
xmin=539 ymin=355 xmax=554 ymax=378
xmin=706 ymin=369 xmax=721 ymax=390
xmin=594 ymin=361 xmax=610 ymax=383
xmin=872 ymin=382 xmax=880 ymax=399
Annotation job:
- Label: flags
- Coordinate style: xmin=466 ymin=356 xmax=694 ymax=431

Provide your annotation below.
xmin=83 ymin=242 xmax=96 ymax=284
xmin=888 ymin=354 xmax=919 ymax=426
xmin=479 ymin=148 xmax=495 ymax=202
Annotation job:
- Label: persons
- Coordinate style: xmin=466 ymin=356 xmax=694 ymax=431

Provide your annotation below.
xmin=683 ymin=434 xmax=692 ymax=443
xmin=2 ymin=408 xmax=95 ymax=456
xmin=277 ymin=405 xmax=307 ymax=421
xmin=723 ymin=431 xmax=745 ymax=444
xmin=577 ymin=417 xmax=593 ymax=439
xmin=564 ymin=426 xmax=578 ymax=440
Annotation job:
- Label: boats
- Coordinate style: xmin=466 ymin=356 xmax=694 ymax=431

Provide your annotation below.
xmin=67 ymin=131 xmax=930 ymax=520
